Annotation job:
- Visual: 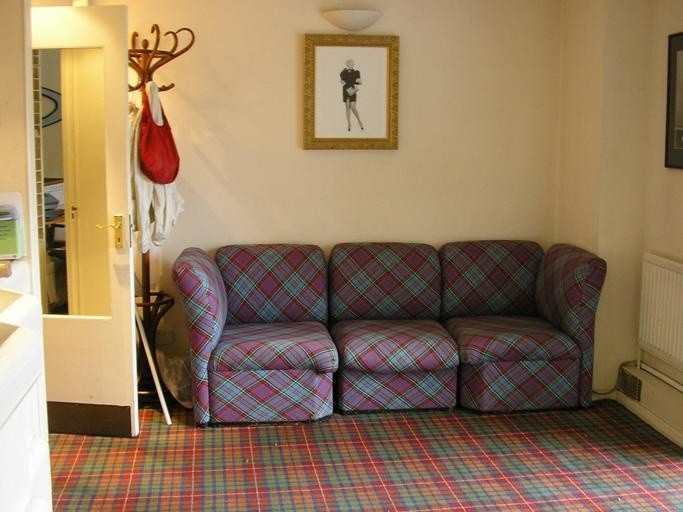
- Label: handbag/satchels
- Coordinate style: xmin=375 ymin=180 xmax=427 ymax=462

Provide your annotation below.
xmin=347 ymin=85 xmax=358 ymax=96
xmin=139 ymin=86 xmax=179 ymax=183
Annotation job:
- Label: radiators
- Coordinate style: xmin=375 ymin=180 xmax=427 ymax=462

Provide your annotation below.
xmin=635 ymin=253 xmax=682 ymax=393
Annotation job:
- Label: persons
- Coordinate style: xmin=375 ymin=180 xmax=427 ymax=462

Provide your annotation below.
xmin=340 ymin=60 xmax=364 ymax=131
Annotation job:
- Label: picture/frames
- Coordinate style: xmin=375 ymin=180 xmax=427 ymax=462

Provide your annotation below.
xmin=304 ymin=33 xmax=400 ymax=153
xmin=665 ymin=32 xmax=683 ymax=168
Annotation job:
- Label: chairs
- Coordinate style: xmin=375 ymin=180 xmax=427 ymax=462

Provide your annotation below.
xmin=172 ymin=243 xmax=340 ymax=428
xmin=441 ymin=238 xmax=608 ymax=413
xmin=328 ymin=241 xmax=461 ymax=414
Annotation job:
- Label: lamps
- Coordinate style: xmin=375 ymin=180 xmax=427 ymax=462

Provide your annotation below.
xmin=323 ymin=8 xmax=385 ymax=31
